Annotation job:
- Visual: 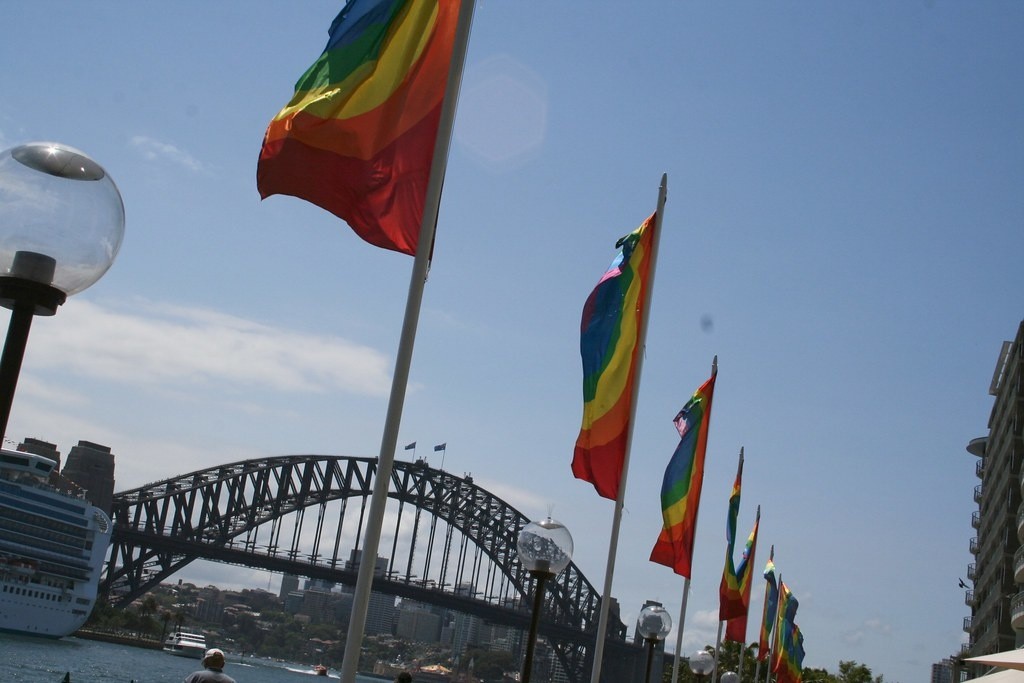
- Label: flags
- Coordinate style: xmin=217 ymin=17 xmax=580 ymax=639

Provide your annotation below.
xmin=769 ymin=581 xmax=805 ymax=683
xmin=650 ymin=373 xmax=720 ymax=578
xmin=725 ymin=518 xmax=760 ymax=643
xmin=434 ymin=443 xmax=446 ymax=450
xmin=257 ymin=0 xmax=462 ymax=259
xmin=719 ymin=461 xmax=743 ymax=620
xmin=406 ymin=441 xmax=416 ymax=449
xmin=570 ymin=211 xmax=659 ymax=504
xmin=758 ymin=558 xmax=778 ymax=662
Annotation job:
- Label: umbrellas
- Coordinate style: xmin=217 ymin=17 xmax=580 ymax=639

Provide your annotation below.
xmin=959 ymin=668 xmax=1024 ymax=683
xmin=962 ymin=648 xmax=1024 ymax=670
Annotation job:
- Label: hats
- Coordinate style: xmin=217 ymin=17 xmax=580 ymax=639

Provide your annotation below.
xmin=203 ymin=648 xmax=226 ymax=663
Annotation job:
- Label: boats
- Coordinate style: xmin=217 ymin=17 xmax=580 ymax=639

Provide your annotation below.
xmin=314 ymin=666 xmax=327 ymax=676
xmin=162 ymin=632 xmax=207 ymax=659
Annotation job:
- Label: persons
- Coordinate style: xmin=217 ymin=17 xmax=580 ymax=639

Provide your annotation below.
xmin=183 ymin=648 xmax=235 ymax=683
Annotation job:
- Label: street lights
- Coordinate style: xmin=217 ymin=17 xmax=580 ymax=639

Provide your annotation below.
xmin=689 ymin=650 xmax=715 ymax=683
xmin=637 ymin=605 xmax=672 ymax=683
xmin=0 ymin=141 xmax=125 ymax=451
xmin=515 ymin=518 xmax=574 ymax=683
